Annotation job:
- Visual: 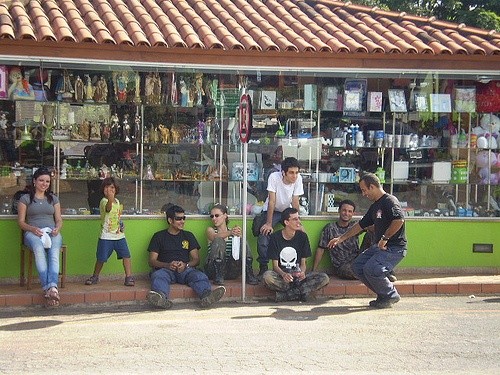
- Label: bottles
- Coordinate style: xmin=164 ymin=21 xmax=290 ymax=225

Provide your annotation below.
xmin=356 ymin=131 xmax=363 ymax=147
xmin=293 ymin=262 xmax=301 ymax=287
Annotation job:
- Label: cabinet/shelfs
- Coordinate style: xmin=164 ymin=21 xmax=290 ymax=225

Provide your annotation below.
xmin=0 ymin=98 xmax=499 ymax=220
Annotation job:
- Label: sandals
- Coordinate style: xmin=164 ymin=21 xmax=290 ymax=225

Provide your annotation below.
xmin=44 ymin=287 xmax=60 ymax=307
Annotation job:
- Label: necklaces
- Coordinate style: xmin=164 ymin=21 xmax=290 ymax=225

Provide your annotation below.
xmin=39 ymin=202 xmax=42 ymax=204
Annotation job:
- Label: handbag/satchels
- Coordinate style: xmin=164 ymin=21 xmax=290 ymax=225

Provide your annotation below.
xmin=12 ymin=185 xmax=53 ymax=214
xmin=251 ymin=212 xmax=264 ymax=237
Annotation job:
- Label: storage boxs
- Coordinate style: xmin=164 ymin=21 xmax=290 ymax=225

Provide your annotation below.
xmin=429 ymin=161 xmax=451 ymax=184
xmin=257 ymin=91 xmax=276 ymax=109
xmin=339 ymin=166 xmax=356 ymax=183
xmin=393 ymin=161 xmax=418 ymax=183
xmin=322 ymin=86 xmax=338 ymax=110
xmin=366 ymin=91 xmax=382 ymax=111
xmin=304 ymin=84 xmax=317 ymax=110
xmin=325 ymin=193 xmax=339 ymax=212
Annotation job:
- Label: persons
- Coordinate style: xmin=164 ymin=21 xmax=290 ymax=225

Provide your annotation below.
xmin=257 ymin=157 xmax=304 ymax=278
xmin=146 ymin=205 xmax=225 ymax=309
xmin=85 ymin=177 xmax=135 ymax=285
xmin=261 ymin=174 xmax=408 ymax=308
xmin=17 ymin=168 xmax=65 ymax=306
xmin=206 ymin=204 xmax=258 ymax=285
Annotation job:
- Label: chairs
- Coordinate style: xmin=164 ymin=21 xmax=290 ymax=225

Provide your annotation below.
xmin=20 ymin=213 xmax=67 ymax=288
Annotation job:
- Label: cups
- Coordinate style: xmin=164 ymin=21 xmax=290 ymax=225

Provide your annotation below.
xmin=368 ymin=130 xmax=410 ymax=148
xmin=450 ymin=132 xmax=498 ymax=148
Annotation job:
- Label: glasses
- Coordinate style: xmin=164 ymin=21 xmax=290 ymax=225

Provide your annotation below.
xmin=210 ymin=214 xmax=224 ymax=218
xmin=171 ymin=216 xmax=186 ymax=221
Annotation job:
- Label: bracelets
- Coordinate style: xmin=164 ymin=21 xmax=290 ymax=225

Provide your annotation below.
xmin=380 ymin=235 xmax=389 ymax=240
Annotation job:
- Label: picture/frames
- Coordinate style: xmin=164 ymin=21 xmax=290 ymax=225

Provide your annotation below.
xmin=453 ymin=85 xmax=478 ymax=113
xmin=388 ymin=89 xmax=407 ymax=112
xmin=415 ymin=94 xmax=429 ymax=112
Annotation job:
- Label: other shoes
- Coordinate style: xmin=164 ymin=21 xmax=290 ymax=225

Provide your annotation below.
xmin=275 ymin=289 xmax=307 ymax=303
xmin=85 ymin=275 xmax=99 ymax=284
xmin=257 ymin=265 xmax=269 ymax=282
xmin=189 ymin=286 xmax=226 ymax=310
xmin=146 ymin=291 xmax=173 ymax=309
xmin=386 ymin=270 xmax=397 ymax=282
xmin=124 ymin=276 xmax=136 ymax=285
xmin=369 ymin=295 xmax=400 ymax=309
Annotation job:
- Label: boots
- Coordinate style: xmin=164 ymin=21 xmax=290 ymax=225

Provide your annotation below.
xmin=247 ymin=257 xmax=258 ymax=285
xmin=214 ymin=258 xmax=225 ymax=285
